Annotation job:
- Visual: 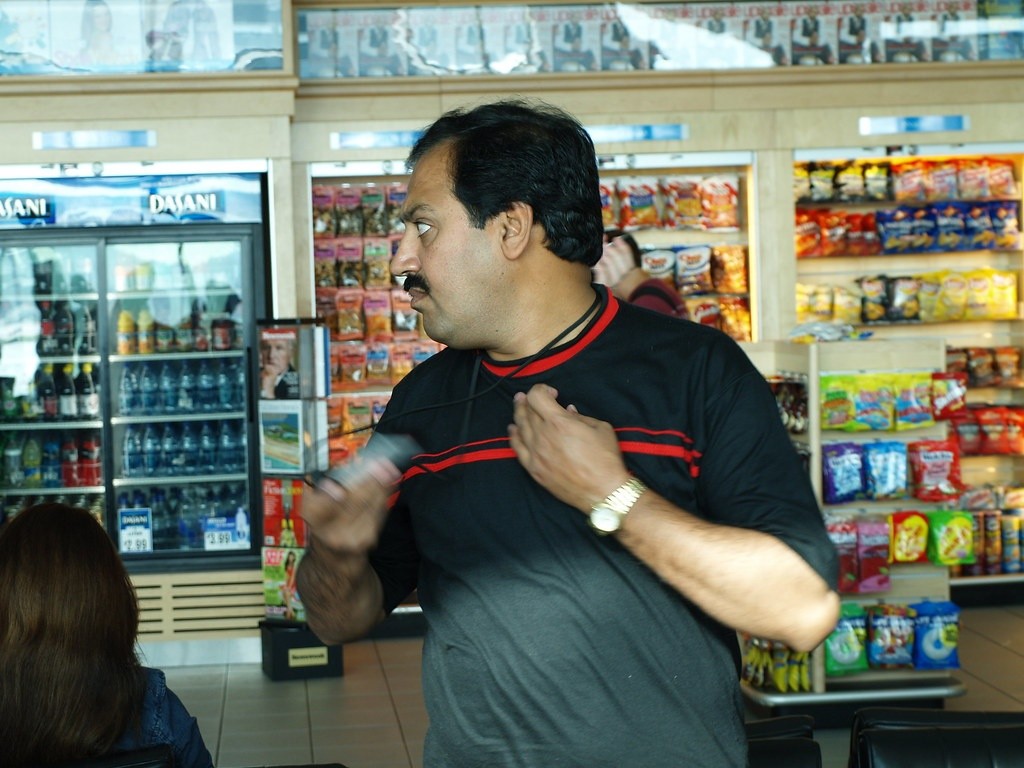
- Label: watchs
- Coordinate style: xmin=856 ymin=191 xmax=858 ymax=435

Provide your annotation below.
xmin=586 ymin=475 xmax=649 ymax=535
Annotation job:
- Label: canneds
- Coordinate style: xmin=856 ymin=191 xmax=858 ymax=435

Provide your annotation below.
xmin=969 ymin=508 xmax=1024 ymax=547
xmin=113 ymin=261 xmax=152 ymax=291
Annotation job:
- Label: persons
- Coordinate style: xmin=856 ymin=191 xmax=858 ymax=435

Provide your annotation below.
xmin=72 ymin=1 xmax=123 ymax=68
xmin=293 ymin=100 xmax=840 ymax=768
xmin=258 ymin=337 xmax=299 ymax=400
xmin=595 ymin=231 xmax=690 ymax=322
xmin=320 ymin=4 xmax=979 ymax=78
xmin=1 ymin=503 xmax=213 ymax=768
xmin=280 ymin=552 xmax=297 ymax=620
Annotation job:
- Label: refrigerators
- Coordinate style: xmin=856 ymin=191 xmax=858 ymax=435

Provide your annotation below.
xmin=0 ymin=156 xmax=277 ymax=667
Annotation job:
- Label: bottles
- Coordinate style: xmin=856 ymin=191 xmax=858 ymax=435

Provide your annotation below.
xmin=0 ymin=253 xmax=247 ymax=547
xmin=235 ymin=507 xmax=247 ymax=546
